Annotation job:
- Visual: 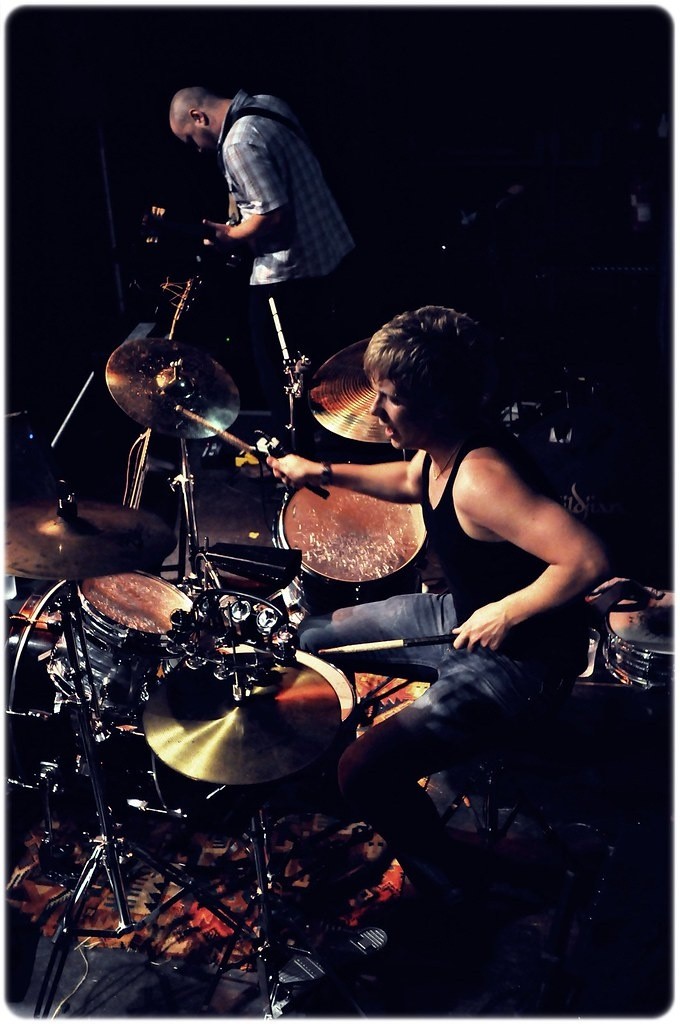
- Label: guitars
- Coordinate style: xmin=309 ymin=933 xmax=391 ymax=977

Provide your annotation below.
xmin=197 ymin=192 xmax=246 ymax=271
xmin=166 ymin=275 xmax=203 ymax=341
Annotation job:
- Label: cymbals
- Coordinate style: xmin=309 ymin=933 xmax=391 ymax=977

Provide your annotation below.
xmin=105 ymin=336 xmax=241 ymax=439
xmin=5 ymin=498 xmax=178 ymax=581
xmin=307 ymin=335 xmax=392 ymax=444
xmin=142 ymin=659 xmax=342 ymax=784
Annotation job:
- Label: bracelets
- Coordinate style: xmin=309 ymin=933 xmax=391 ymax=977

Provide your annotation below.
xmin=321 ymin=459 xmax=333 ymax=486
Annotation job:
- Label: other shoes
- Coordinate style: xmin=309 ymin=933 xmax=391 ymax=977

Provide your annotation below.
xmin=366 ymin=867 xmax=481 ymax=939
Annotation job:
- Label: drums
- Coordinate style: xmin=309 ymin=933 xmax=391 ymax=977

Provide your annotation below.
xmin=215 ymin=641 xmax=357 ymax=725
xmin=601 ymin=590 xmax=673 ymax=690
xmin=272 ymin=483 xmax=431 ymax=619
xmin=45 ymin=570 xmax=194 ymax=717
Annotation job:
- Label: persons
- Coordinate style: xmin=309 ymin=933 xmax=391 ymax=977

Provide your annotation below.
xmin=169 ymin=83 xmax=356 ymax=333
xmin=287 ymin=303 xmax=608 ymax=949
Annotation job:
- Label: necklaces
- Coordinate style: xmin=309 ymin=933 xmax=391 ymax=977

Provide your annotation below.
xmin=432 ymin=443 xmax=459 ymax=482
